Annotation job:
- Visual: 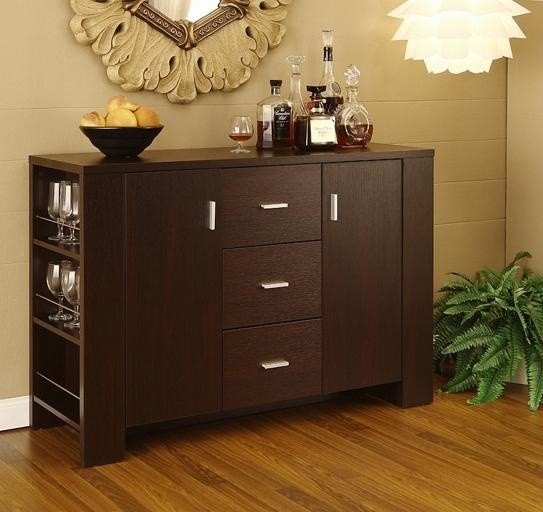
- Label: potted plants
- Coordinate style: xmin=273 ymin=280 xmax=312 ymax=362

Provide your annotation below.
xmin=432 ymin=251 xmax=543 ymax=411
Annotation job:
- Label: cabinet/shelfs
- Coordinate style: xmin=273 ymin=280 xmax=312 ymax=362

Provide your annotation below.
xmin=28 ymin=142 xmax=433 ymax=467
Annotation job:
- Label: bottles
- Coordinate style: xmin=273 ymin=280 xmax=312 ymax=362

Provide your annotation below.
xmin=333 ymin=63 xmax=374 ymax=148
xmin=318 ymin=25 xmax=344 ymax=116
xmin=284 ymin=54 xmax=309 ymax=148
xmin=295 ymin=84 xmax=338 ymax=152
xmin=254 ymin=78 xmax=292 ymax=152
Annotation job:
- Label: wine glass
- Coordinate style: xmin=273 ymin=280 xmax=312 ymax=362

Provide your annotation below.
xmin=228 ymin=114 xmax=254 ymax=154
xmin=43 ymin=175 xmax=71 ymax=241
xmin=44 ymin=259 xmax=73 ymax=322
xmin=57 ymin=180 xmax=82 ymax=246
xmin=60 ymin=266 xmax=81 ymax=331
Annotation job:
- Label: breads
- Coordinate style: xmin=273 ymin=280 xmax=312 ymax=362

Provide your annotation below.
xmin=80 ymin=95 xmax=161 ymax=127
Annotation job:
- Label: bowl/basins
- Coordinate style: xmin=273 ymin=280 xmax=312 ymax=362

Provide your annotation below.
xmin=79 ymin=123 xmax=165 ymax=161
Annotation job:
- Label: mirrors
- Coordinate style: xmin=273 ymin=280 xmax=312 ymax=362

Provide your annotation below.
xmin=144 ymin=0 xmax=221 ymax=23
